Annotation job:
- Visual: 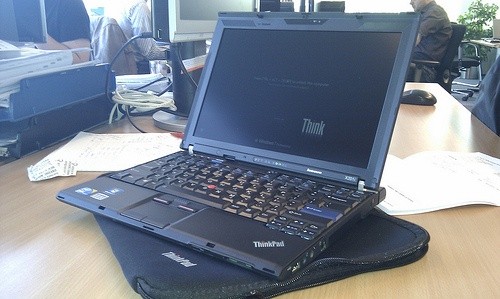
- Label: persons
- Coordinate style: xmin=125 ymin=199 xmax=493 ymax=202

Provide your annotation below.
xmin=0 ymin=0 xmax=91 ymax=64
xmin=119 ymin=0 xmax=171 ymax=74
xmin=405 ymin=0 xmax=453 ymax=82
xmin=468 ymin=55 xmax=500 ymax=137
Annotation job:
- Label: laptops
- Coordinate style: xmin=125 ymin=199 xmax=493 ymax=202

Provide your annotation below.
xmin=53 ymin=10 xmax=424 ymax=280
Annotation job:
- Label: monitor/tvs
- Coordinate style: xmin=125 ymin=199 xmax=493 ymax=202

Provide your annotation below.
xmin=148 ymin=1 xmax=259 ymax=72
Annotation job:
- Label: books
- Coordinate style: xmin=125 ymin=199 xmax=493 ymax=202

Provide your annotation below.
xmin=0 ymin=39 xmax=73 ymax=108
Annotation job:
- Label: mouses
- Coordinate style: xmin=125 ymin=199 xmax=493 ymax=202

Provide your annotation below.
xmin=401 ymin=87 xmax=437 ymax=107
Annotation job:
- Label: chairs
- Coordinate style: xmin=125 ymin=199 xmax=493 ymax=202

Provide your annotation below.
xmin=450 ymin=22 xmax=481 ymax=93
xmin=411 ymin=24 xmax=468 ymax=82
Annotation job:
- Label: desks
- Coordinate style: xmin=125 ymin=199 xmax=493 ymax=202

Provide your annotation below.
xmin=0 ymin=83 xmax=500 ymax=299
xmin=470 ymin=40 xmax=500 ymax=48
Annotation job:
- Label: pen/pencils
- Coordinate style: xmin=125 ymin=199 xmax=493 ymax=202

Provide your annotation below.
xmin=171 ymin=132 xmax=184 ymax=138
